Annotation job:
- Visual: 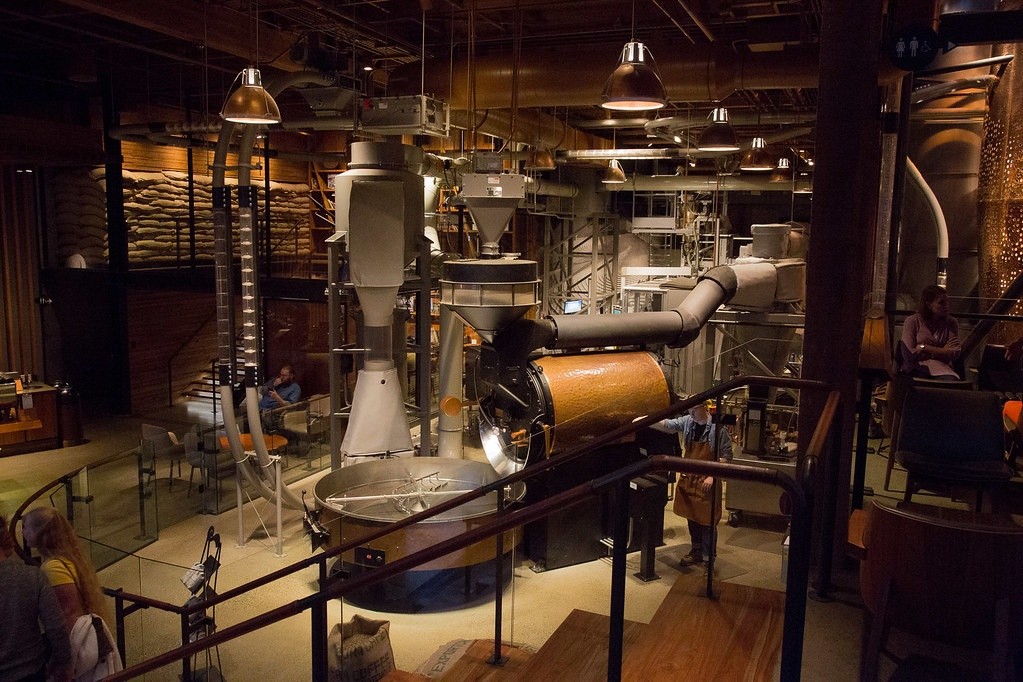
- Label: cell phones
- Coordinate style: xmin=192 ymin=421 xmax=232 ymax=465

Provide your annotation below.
xmin=268 ymin=387 xmax=273 ymax=392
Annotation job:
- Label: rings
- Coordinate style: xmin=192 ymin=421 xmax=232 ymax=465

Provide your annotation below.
xmin=948 ymin=344 xmax=952 ymax=346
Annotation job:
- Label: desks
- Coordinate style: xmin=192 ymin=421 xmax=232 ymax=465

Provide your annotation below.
xmin=897 ymin=503 xmax=1013 ymax=527
xmin=220 ymin=432 xmax=288 ymax=466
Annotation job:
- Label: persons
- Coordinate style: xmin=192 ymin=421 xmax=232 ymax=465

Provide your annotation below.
xmin=1004 ymin=336 xmax=1023 ymax=360
xmin=632 ymin=394 xmax=733 ymax=569
xmin=0 ymin=504 xmax=115 ymax=682
xmin=257 ymin=365 xmax=301 ymax=434
xmin=900 ymin=284 xmax=962 ymax=380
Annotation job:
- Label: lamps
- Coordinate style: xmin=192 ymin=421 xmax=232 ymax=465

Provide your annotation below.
xmin=793 ymin=148 xmax=814 ymax=193
xmin=523 ymin=107 xmax=555 ymax=170
xmin=601 ymin=128 xmax=626 ymax=184
xmin=220 ymin=1 xmax=282 ymax=124
xmin=769 ymin=141 xmax=799 ymax=182
xmin=739 ymin=90 xmax=775 ymax=171
xmin=698 ymin=105 xmax=739 ymax=152
xmin=601 ymin=0 xmax=669 ymax=110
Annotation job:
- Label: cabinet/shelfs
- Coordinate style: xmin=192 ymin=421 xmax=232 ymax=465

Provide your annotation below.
xmin=0 ymin=379 xmax=59 ymax=458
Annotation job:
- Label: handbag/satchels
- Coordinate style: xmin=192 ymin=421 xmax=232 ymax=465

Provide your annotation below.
xmin=41 ymin=618 xmax=112 ymax=666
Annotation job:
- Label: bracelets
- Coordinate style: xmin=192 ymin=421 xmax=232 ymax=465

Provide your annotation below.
xmin=919 ymin=343 xmax=925 ymax=351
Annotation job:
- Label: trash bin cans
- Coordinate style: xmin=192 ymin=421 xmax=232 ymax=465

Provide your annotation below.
xmin=53 ymin=379 xmax=81 ymax=447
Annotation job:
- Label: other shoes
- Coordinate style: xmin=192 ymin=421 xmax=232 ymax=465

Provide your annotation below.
xmin=680 ymin=548 xmax=703 ymax=566
xmin=686 ymin=558 xmax=715 ymax=574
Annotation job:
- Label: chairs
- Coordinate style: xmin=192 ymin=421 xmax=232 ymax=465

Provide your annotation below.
xmin=239 ymin=386 xmax=263 ymax=415
xmin=141 ymin=423 xmax=186 ymax=492
xmin=184 ymin=433 xmax=239 ymax=501
xmin=859 ymin=496 xmax=1022 ymax=682
xmin=285 ymin=395 xmax=331 ymax=461
xmin=882 ymin=339 xmax=1023 ymax=509
